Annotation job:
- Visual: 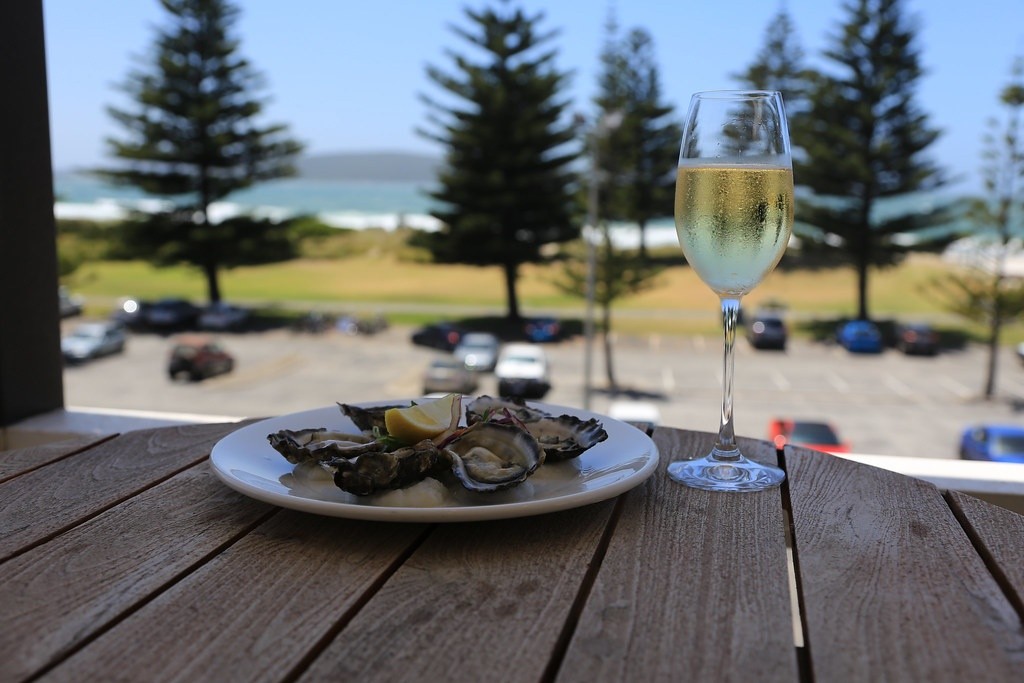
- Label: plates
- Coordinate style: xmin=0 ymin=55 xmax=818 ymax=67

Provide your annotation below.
xmin=205 ymin=395 xmax=660 ymax=523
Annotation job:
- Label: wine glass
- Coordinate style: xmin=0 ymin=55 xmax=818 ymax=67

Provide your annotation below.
xmin=666 ymin=87 xmax=795 ymax=494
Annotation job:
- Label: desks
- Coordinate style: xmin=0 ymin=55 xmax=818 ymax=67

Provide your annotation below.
xmin=0 ymin=419 xmax=1024 ymax=683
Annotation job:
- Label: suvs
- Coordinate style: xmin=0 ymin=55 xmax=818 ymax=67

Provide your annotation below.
xmin=747 ymin=316 xmax=786 ymax=349
xmin=495 ymin=342 xmax=549 ymax=394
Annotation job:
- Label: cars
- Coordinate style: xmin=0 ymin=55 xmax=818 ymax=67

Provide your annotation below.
xmin=769 ymin=419 xmax=845 ymax=454
xmin=61 ymin=322 xmax=123 ymax=362
xmin=116 ymin=299 xmax=198 ymax=335
xmin=199 ymin=305 xmax=249 ymax=329
xmin=451 ymin=334 xmax=499 ymax=371
xmin=961 ymin=426 xmax=1024 ymax=463
xmin=169 ymin=340 xmax=234 ymax=378
xmin=835 ymin=320 xmax=883 ymax=353
xmin=58 ymin=294 xmax=80 ymax=316
xmin=424 ymin=358 xmax=475 ymax=394
xmin=897 ymin=321 xmax=939 ymax=354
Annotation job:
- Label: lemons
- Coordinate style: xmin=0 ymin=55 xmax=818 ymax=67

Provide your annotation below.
xmin=384 ymin=393 xmax=455 ymax=441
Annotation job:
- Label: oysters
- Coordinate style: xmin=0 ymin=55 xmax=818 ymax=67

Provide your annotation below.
xmin=267 ymin=393 xmax=609 ymax=497
